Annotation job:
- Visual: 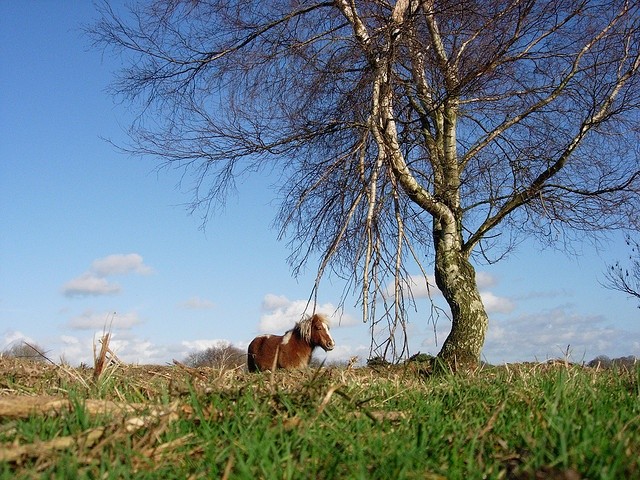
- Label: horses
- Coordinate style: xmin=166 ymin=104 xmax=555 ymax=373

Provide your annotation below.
xmin=247 ymin=313 xmax=336 ymax=374
